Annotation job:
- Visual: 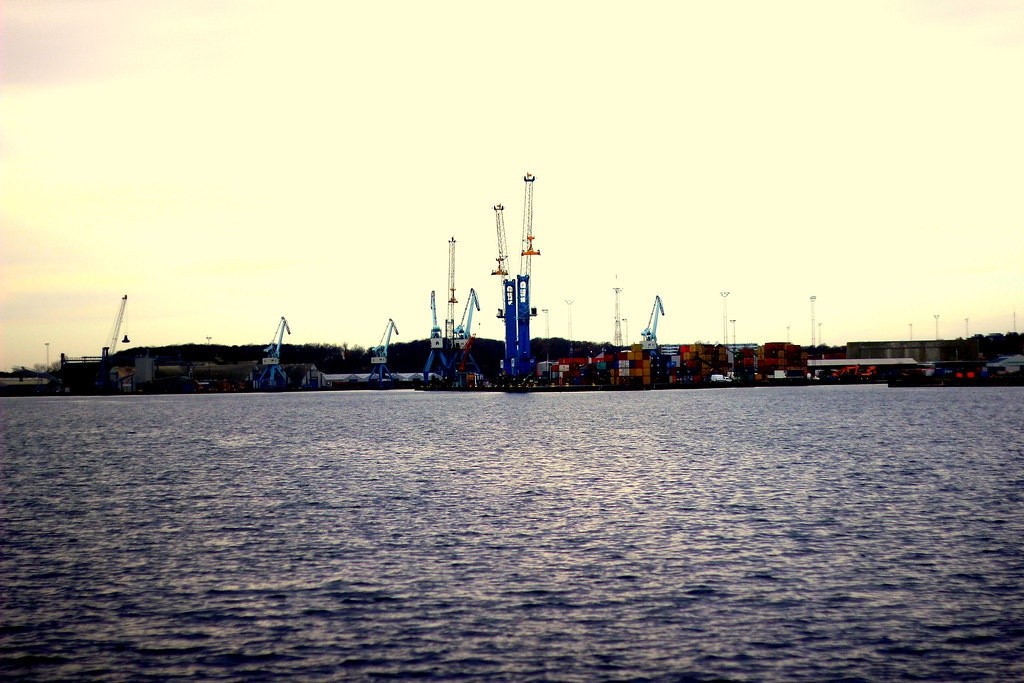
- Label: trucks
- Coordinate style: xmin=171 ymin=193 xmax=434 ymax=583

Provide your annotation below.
xmin=711 ymin=375 xmax=729 ymax=382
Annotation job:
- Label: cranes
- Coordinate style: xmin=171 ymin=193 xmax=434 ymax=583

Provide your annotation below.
xmin=447 ymin=235 xmax=483 ymax=381
xmin=366 ymin=319 xmax=399 ymax=385
xmin=95 ymin=294 xmax=128 ymax=379
xmin=641 ymin=295 xmax=665 ymax=364
xmin=492 ymin=202 xmax=517 ymax=376
xmin=423 ymin=291 xmax=452 ymax=383
xmin=253 ymin=317 xmax=291 ymax=387
xmin=517 ymin=172 xmax=540 ymax=376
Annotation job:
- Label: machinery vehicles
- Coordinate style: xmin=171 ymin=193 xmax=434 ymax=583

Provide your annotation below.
xmin=830 ymin=365 xmax=876 ymax=382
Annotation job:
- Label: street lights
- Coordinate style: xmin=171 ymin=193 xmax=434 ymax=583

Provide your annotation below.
xmin=541 ymin=307 xmax=550 ymax=338
xmin=719 ymin=292 xmax=728 ymax=343
xmin=908 ymin=324 xmax=913 ymax=340
xmin=786 ymin=326 xmax=790 ymax=342
xmin=933 ymin=314 xmax=940 ymax=339
xmin=564 ymin=298 xmax=574 ymax=341
xmin=44 ymin=342 xmax=50 ymax=372
xmin=206 ymin=336 xmax=212 ymax=345
xmin=730 ymin=319 xmax=737 ymax=344
xmin=965 ymin=316 xmax=969 ymax=338
xmin=818 ymin=322 xmax=823 ymax=346
xmin=809 ymin=295 xmax=818 ymax=347
